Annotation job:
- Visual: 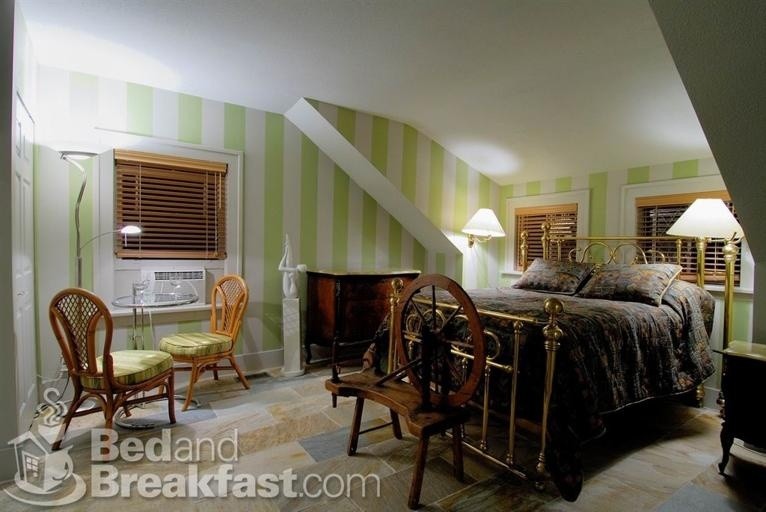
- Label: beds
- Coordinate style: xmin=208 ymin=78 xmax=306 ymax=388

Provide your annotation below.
xmin=388 ymin=220 xmax=717 ymax=503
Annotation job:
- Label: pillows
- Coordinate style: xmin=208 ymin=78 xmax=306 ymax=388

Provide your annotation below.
xmin=510 ymin=257 xmax=596 ymax=296
xmin=575 ymin=263 xmax=683 ymax=307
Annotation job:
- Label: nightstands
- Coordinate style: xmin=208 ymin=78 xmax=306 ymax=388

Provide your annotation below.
xmin=712 ymin=340 xmax=766 ymax=474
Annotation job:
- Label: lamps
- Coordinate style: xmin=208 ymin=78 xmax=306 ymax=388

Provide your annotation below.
xmin=57 ymin=150 xmax=143 ymax=418
xmin=664 ymin=198 xmax=745 ymax=243
xmin=461 ymin=208 xmax=507 ymax=249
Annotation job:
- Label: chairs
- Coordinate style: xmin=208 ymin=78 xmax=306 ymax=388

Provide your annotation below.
xmin=50 ymin=287 xmax=176 ymax=461
xmin=158 ymin=273 xmax=251 ymax=411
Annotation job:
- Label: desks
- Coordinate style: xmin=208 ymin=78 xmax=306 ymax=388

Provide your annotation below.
xmin=113 ymin=294 xmax=202 ymax=430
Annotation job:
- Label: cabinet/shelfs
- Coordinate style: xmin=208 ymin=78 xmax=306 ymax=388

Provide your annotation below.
xmin=303 ymin=270 xmax=423 ymax=369
